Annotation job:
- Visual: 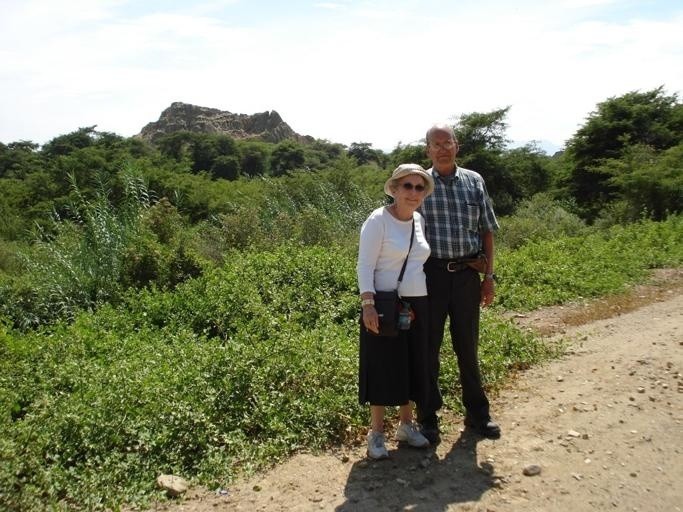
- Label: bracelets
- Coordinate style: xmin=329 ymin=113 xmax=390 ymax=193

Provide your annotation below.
xmin=362 ymin=299 xmax=374 ymax=306
xmin=485 ymin=273 xmax=493 ymax=279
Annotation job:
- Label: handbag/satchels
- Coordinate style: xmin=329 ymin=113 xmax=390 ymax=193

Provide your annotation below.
xmin=373 ymin=290 xmax=398 ymax=323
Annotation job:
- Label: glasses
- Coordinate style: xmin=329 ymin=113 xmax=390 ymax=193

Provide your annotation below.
xmin=430 ymin=143 xmax=451 ymax=151
xmin=400 ymin=182 xmax=425 ymax=192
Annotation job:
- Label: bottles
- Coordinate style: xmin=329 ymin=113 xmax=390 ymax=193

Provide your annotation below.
xmin=398 ymin=304 xmax=411 ymax=331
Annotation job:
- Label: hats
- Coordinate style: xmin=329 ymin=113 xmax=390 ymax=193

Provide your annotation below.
xmin=384 ymin=163 xmax=434 ymax=198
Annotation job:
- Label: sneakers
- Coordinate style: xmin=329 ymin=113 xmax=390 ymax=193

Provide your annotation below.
xmin=395 ymin=421 xmax=440 ymax=446
xmin=465 ymin=415 xmax=500 ymax=435
xmin=367 ymin=431 xmax=389 ymax=460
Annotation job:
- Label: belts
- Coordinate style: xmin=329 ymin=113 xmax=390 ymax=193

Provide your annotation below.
xmin=425 ymin=257 xmax=470 ymax=272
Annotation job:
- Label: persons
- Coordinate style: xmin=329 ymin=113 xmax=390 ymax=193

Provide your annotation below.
xmin=414 ymin=124 xmax=501 ymax=437
xmin=357 ymin=163 xmax=431 ymax=458
